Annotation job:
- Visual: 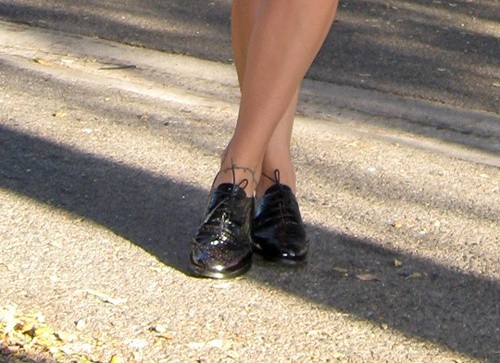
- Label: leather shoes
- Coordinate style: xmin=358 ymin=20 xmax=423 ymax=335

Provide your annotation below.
xmin=249 ymin=168 xmax=310 ymax=264
xmin=190 ymin=157 xmax=254 ymax=279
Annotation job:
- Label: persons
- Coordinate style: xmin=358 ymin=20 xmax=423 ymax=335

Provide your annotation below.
xmin=190 ymin=0 xmax=339 ymax=279
